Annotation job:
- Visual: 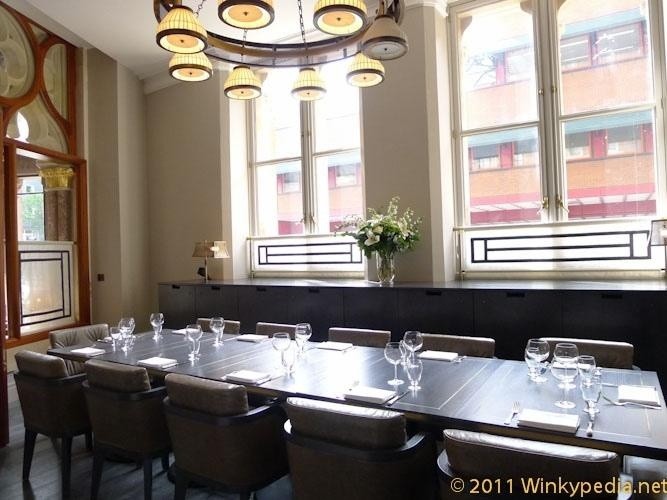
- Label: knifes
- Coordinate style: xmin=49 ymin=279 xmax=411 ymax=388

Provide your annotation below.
xmin=224 ymin=334 xmax=241 ymax=341
xmin=597 ymin=382 xmax=656 ymax=390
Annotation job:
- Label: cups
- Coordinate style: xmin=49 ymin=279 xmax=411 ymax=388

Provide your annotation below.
xmin=272 ymin=333 xmax=290 ymax=371
xmin=398 ymin=340 xmax=409 ymax=364
xmin=150 ymin=313 xmax=164 ymax=341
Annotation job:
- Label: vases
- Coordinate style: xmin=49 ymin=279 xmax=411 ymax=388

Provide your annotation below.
xmin=376 ymin=249 xmax=395 ymax=285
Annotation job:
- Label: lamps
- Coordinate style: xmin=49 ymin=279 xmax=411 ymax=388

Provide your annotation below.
xmin=153 ymin=0 xmax=408 ymax=101
xmin=192 ymin=240 xmax=231 ymax=283
xmin=35 ymin=160 xmax=76 ymax=193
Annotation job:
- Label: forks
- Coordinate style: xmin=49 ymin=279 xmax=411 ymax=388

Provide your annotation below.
xmin=504 ymin=401 xmax=520 ymax=425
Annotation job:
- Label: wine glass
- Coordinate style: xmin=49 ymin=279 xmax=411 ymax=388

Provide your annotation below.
xmin=553 ymin=342 xmax=578 ymax=383
xmin=294 ymin=323 xmax=313 ymax=353
xmin=110 ymin=318 xmax=135 ymax=351
xmin=550 ymin=356 xmax=578 ymax=410
xmin=580 ymin=375 xmax=603 ymax=414
xmin=403 ymin=331 xmax=422 ymax=369
xmin=185 ymin=325 xmax=203 ymax=358
xmin=524 ymin=339 xmax=550 ymax=383
xmin=208 ymin=318 xmax=226 ymax=345
xmin=575 ymin=354 xmax=595 ymax=388
xmin=383 ymin=343 xmax=407 ymax=385
xmin=406 ymin=359 xmax=425 ymax=391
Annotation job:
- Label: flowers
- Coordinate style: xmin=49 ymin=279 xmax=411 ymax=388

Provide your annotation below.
xmin=333 ymin=193 xmax=424 ymax=271
